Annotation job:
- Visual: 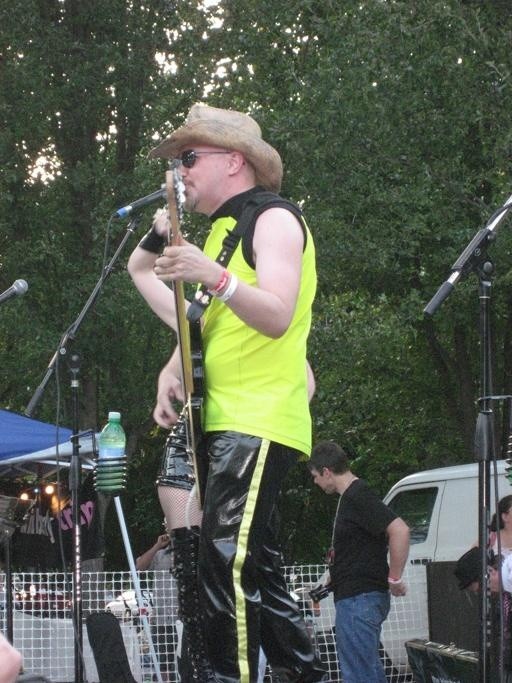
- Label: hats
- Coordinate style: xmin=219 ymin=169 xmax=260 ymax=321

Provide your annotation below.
xmin=146 ymin=104 xmax=284 ymax=192
xmin=454 ymin=547 xmax=490 ymax=589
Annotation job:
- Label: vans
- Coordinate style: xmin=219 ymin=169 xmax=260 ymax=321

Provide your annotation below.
xmin=305 ymin=455 xmax=512 ymax=682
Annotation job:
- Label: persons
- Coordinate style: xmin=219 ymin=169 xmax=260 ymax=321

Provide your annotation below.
xmin=306 ymin=440 xmax=409 ymax=682
xmin=149 ymin=99 xmax=329 ymax=680
xmin=452 ymin=546 xmax=511 ymax=597
xmin=473 ymin=493 xmax=511 ymax=682
xmin=124 ymin=200 xmax=316 ymax=681
xmin=137 ymin=516 xmax=185 ymax=681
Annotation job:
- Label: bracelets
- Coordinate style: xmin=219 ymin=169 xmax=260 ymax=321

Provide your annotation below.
xmin=206 ymin=265 xmax=229 ymax=296
xmin=140 ymin=225 xmax=166 ymax=254
xmin=217 ymin=273 xmax=238 ymax=302
xmin=387 ymin=577 xmax=402 ymax=584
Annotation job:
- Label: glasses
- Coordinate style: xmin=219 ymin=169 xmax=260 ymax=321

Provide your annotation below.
xmin=179 ymin=148 xmax=231 ymax=168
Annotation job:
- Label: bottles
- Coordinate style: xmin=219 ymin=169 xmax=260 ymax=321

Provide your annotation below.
xmin=97 ymin=410 xmax=128 ymax=493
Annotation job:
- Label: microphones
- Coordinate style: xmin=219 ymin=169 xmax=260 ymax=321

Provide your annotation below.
xmin=0 ymin=279 xmax=28 ymax=304
xmin=112 ymin=178 xmax=179 ymax=221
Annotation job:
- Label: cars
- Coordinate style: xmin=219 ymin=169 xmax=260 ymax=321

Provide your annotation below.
xmin=103 ymin=588 xmax=153 ymax=622
xmin=11 ymin=584 xmax=72 ymax=619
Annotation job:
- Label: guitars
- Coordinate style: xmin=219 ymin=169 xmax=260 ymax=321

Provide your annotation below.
xmin=166 ymin=169 xmax=208 ymax=509
